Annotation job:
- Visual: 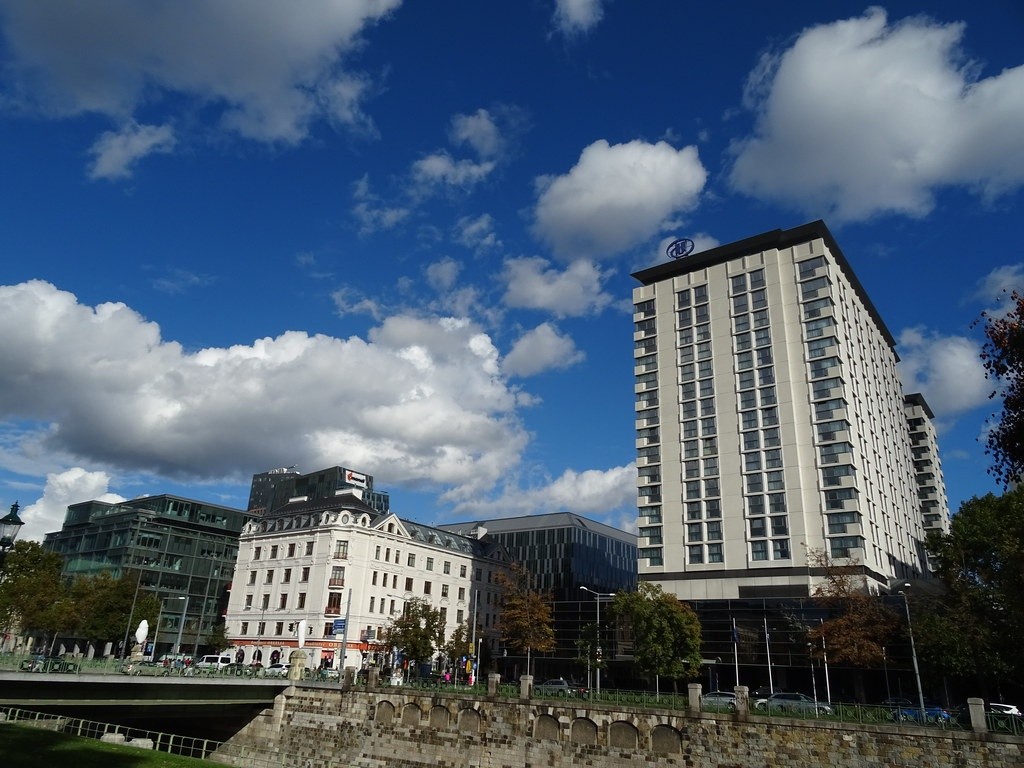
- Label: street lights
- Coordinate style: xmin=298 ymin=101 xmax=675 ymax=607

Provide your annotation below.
xmin=808 ymin=641 xmax=817 ymax=718
xmin=246 ymin=605 xmax=280 ymax=664
xmin=150 ymin=597 xmax=185 ymax=662
xmin=387 ymin=593 xmax=428 ymax=632
xmin=476 ymin=638 xmax=482 ymax=686
xmin=579 ymin=585 xmax=618 ymax=703
xmin=49 ymin=601 xmax=76 ymax=655
xmin=898 ymin=590 xmax=927 ymax=723
xmin=118 ymin=527 xmax=168 ymax=671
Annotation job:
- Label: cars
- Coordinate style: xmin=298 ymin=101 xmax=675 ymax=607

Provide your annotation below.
xmin=357 ymin=668 xmax=384 ymax=685
xmin=452 ymin=672 xmax=471 ymax=685
xmin=751 ymin=687 xmax=784 ymax=698
xmin=122 ymin=659 xmax=169 ymax=677
xmin=817 ymin=692 xmax=875 ymax=718
xmin=221 ymin=661 xmax=253 ymax=677
xmin=879 ymin=695 xmax=938 ymax=722
xmin=990 ymin=703 xmax=1022 ymax=729
xmin=700 ymin=692 xmax=736 ymax=712
xmin=950 ymin=704 xmax=971 ymax=726
xmin=265 ymin=663 xmax=290 ymax=678
xmin=326 ymin=668 xmax=338 ymax=680
xmin=21 ymin=655 xmax=81 ymax=673
xmin=382 ymin=667 xmax=432 ymax=688
xmin=244 ymin=663 xmax=265 ymax=677
xmin=755 ymin=698 xmax=766 ymax=711
xmin=893 ymin=701 xmax=956 ymax=724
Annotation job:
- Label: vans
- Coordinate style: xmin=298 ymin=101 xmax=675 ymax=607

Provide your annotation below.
xmin=156 ymin=653 xmax=196 ymax=673
xmin=194 ymin=655 xmax=231 ymax=673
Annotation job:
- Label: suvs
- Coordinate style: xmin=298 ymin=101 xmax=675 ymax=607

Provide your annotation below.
xmin=534 ymin=679 xmax=569 ymax=696
xmin=767 ymin=692 xmax=834 ymax=717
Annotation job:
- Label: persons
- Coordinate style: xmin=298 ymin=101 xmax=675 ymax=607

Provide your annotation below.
xmin=433 ymin=676 xmax=442 ymax=688
xmin=444 ymin=671 xmax=451 ymax=688
xmin=311 ymin=665 xmax=328 ymax=683
xmin=207 ymin=660 xmax=215 ymax=678
xmin=162 ymin=656 xmax=198 ymax=677
